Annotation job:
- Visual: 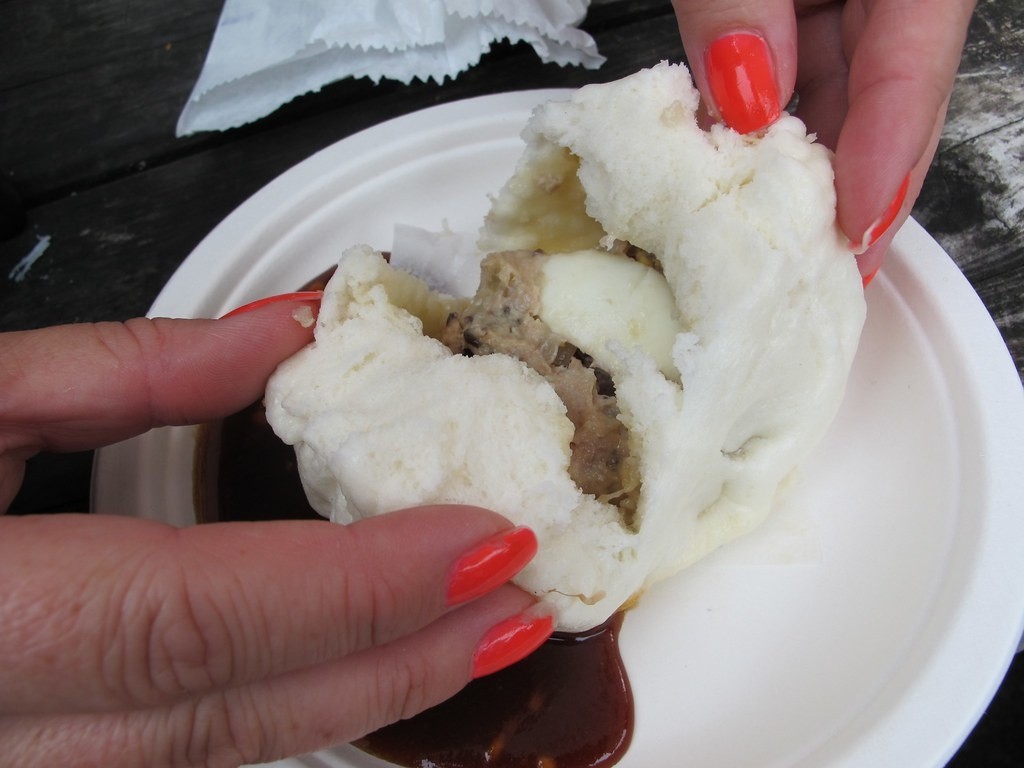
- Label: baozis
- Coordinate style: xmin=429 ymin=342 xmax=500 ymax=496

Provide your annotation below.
xmin=264 ymin=58 xmax=868 ymax=637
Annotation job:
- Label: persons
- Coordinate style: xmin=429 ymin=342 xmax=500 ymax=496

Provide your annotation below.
xmin=0 ymin=0 xmax=976 ymax=768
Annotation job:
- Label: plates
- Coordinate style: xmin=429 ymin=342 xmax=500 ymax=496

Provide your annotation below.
xmin=92 ymin=85 xmax=1024 ymax=768
